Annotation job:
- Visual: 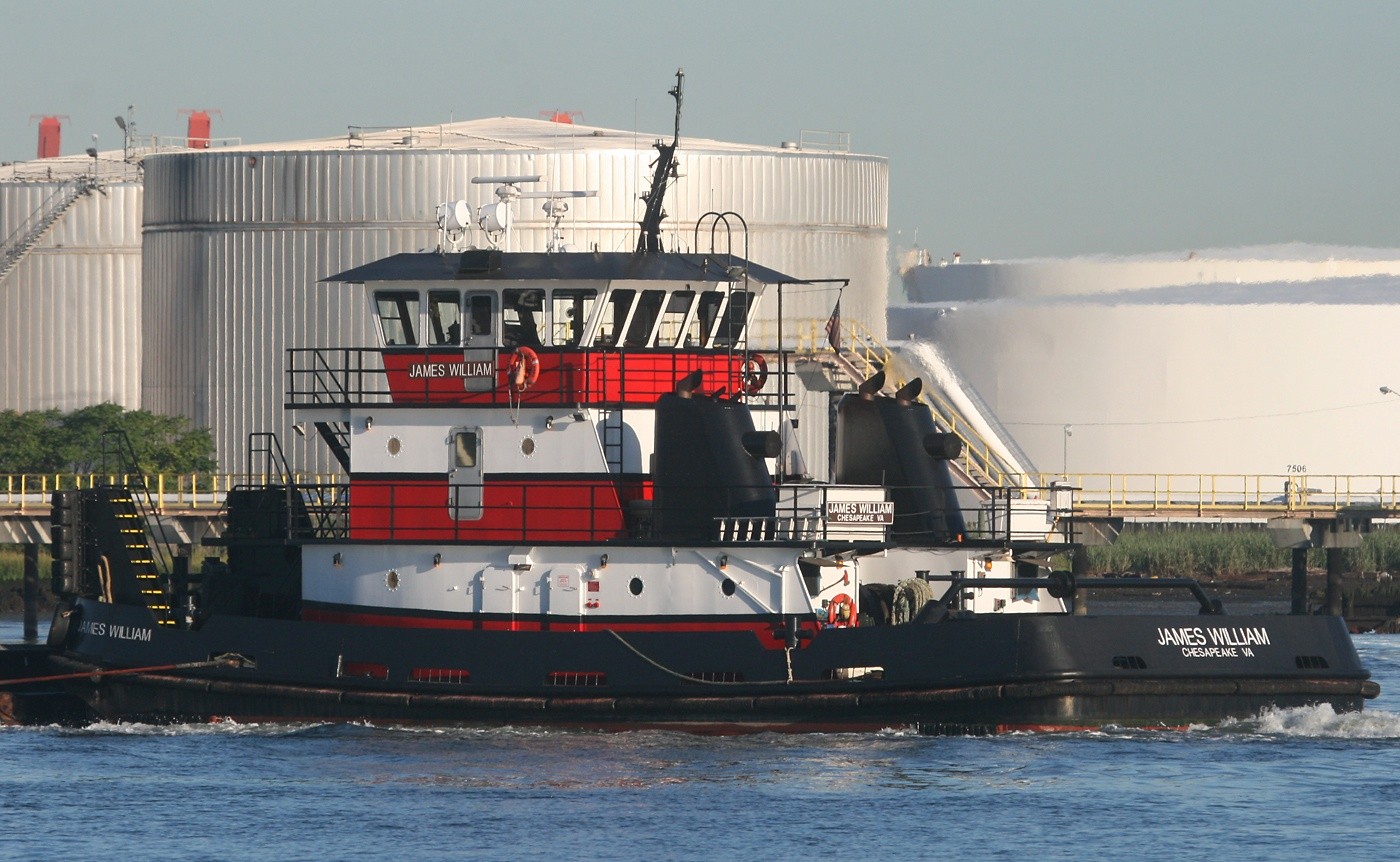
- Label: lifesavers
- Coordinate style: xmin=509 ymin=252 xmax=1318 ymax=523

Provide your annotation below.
xmin=505 ymin=345 xmax=540 ymax=393
xmin=828 ymin=593 xmax=857 ymax=629
xmin=740 ymin=353 xmax=768 ymax=395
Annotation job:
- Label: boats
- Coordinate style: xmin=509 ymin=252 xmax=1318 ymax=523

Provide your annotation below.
xmin=0 ymin=66 xmax=1382 ymax=734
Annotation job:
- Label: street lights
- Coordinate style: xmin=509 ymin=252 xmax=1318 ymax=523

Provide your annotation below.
xmin=1063 ymin=424 xmax=1071 ymax=477
xmin=86 ymin=148 xmax=97 ymax=178
xmin=114 ymin=116 xmax=126 ymax=160
